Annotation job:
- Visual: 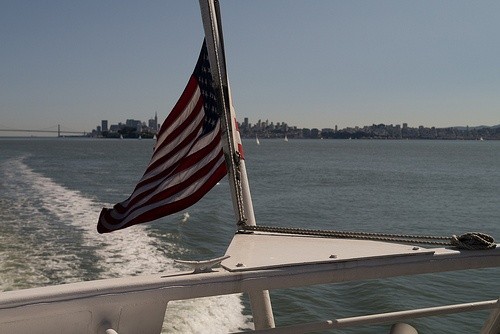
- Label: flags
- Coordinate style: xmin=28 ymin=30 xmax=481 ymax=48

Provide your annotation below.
xmin=98 ymin=35 xmax=246 ymax=233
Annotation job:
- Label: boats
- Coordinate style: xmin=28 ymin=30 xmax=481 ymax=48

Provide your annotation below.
xmin=102 ymin=126 xmax=154 ymax=140
